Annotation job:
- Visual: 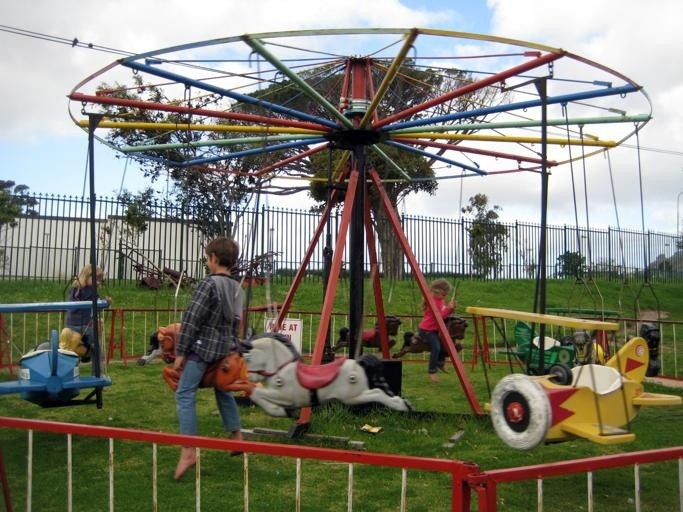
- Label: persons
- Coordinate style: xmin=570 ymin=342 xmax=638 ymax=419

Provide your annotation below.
xmin=63 ymin=264 xmax=112 ymax=362
xmin=418 ymin=278 xmax=457 ymax=383
xmin=173 ymin=238 xmax=246 ymax=482
